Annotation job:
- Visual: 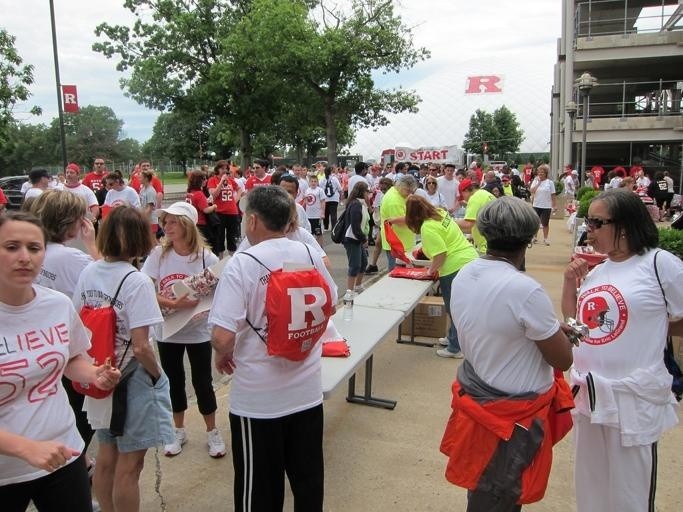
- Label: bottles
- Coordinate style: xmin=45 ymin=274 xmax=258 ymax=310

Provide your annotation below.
xmin=342 ymin=289 xmax=354 ymax=323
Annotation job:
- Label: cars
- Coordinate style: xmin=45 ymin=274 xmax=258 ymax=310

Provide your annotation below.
xmin=0 ymin=174 xmax=30 ymax=211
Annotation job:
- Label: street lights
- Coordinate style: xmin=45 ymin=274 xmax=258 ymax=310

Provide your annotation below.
xmin=575 ymin=72 xmax=597 ymax=185
xmin=565 ymin=100 xmax=577 ymax=168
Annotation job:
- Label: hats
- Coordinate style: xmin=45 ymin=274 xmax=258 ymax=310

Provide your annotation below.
xmin=155 ymin=202 xmax=198 ymax=224
xmin=458 ymin=180 xmax=471 ymax=201
xmin=67 ymin=164 xmax=80 ymax=174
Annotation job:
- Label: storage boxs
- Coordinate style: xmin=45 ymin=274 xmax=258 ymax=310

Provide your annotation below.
xmin=400 ymin=296 xmax=447 ymax=338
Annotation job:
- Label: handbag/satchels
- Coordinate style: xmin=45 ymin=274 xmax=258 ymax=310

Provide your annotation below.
xmin=330 ymin=212 xmax=348 ymax=243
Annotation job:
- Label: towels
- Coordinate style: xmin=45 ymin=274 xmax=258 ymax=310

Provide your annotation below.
xmin=389 ymin=267 xmax=439 ymax=280
xmin=384 ymin=220 xmax=411 ymax=263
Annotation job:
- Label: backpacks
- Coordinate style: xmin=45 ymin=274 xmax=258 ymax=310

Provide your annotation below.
xmin=236 ymin=243 xmax=332 ymax=362
xmin=323 ymin=178 xmax=336 ymax=198
xmin=72 ymin=271 xmax=135 ymax=399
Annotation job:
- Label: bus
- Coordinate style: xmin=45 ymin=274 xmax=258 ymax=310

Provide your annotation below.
xmin=488 ymin=160 xmax=506 ymax=171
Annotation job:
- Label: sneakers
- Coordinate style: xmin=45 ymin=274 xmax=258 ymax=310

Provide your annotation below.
xmin=440 ymin=337 xmax=451 ymax=346
xmin=365 ymin=265 xmax=378 ymax=275
xmin=544 ymin=240 xmax=550 ymax=245
xmin=353 ymin=285 xmax=364 ymax=293
xmin=165 ymin=427 xmax=188 ymax=456
xmin=437 ymin=348 xmax=464 ymax=358
xmin=207 ymin=428 xmax=226 ymax=458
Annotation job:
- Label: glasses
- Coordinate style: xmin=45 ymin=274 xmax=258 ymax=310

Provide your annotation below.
xmin=584 ymin=216 xmax=613 ymax=228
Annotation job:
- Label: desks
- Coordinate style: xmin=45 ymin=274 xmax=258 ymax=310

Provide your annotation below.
xmin=348 ymin=267 xmax=435 ymax=405
xmin=323 ymin=302 xmax=406 ymax=409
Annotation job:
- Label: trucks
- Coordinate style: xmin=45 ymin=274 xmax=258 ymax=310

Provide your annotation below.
xmin=378 ymin=145 xmax=465 ymax=170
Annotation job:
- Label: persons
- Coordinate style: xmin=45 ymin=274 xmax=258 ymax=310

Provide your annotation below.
xmin=28 ymin=190 xmax=104 ymax=512
xmin=71 ymin=206 xmax=176 ymax=512
xmin=559 ymin=166 xmax=673 ymax=221
xmin=405 ymin=193 xmax=480 ymax=358
xmin=562 ymin=186 xmax=682 ymax=512
xmin=1 ymin=211 xmax=123 ymax=512
xmin=207 ymin=183 xmax=339 ymax=512
xmin=140 ymin=201 xmax=227 ymax=458
xmin=448 ymin=195 xmax=573 ymax=512
xmin=21 ymin=157 xmax=165 ymax=243
xmin=185 ymin=158 xmax=556 ymax=293
